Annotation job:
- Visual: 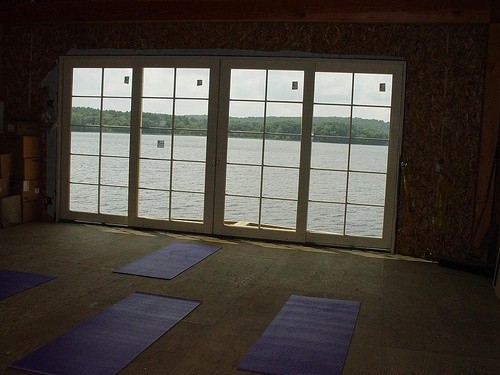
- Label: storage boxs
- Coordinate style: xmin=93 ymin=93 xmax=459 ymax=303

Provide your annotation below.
xmin=0 ymin=70 xmax=54 ymax=229
